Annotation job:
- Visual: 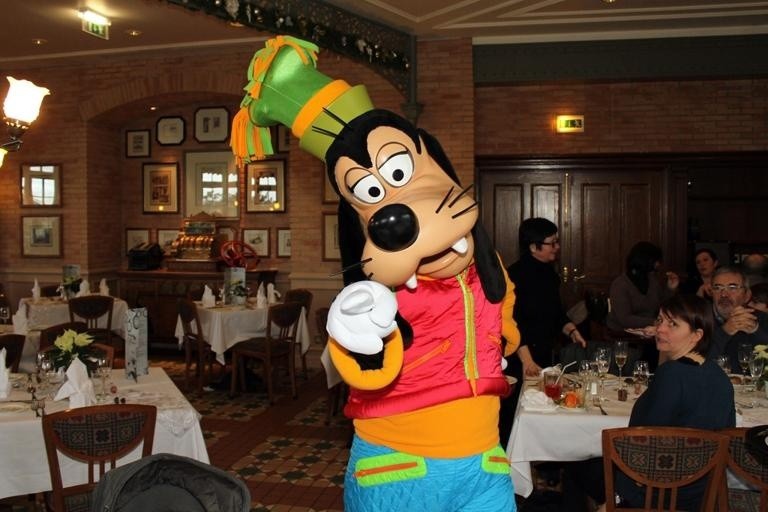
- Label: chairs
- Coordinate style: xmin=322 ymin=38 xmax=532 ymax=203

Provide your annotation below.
xmin=315 ymin=307 xmax=349 ymax=427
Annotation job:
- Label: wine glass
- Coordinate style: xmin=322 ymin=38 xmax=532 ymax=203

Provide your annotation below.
xmin=714 ymin=345 xmax=765 ymax=409
xmin=27 ymin=349 xmax=113 ymax=416
xmin=578 ymin=341 xmax=651 ymax=401
xmin=217 ymin=287 xmax=240 ymax=311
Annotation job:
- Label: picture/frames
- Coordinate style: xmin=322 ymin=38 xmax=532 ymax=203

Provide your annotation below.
xmin=18 ymin=161 xmax=64 ymax=260
xmin=321 ymin=162 xmax=341 ymax=262
xmin=124 ymin=105 xmax=290 ymax=258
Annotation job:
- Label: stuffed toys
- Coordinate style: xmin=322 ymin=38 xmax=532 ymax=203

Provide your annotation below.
xmin=228 ymin=30 xmax=523 ymax=512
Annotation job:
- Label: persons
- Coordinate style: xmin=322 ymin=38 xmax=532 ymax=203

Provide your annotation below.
xmin=605 ymin=239 xmax=680 ymax=356
xmin=681 ymin=249 xmax=719 ymax=302
xmin=498 ymin=215 xmax=587 ymax=458
xmin=696 ymin=264 xmax=768 ymax=378
xmin=562 ymin=292 xmax=737 ymax=511
xmin=741 ymin=253 xmax=768 ymax=305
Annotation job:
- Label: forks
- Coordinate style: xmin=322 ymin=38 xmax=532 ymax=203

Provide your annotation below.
xmin=591 ymin=396 xmax=609 ymax=416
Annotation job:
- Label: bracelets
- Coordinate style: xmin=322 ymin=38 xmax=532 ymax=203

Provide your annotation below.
xmin=568 ymin=328 xmax=577 ymax=336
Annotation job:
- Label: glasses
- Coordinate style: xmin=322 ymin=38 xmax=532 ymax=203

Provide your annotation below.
xmin=712 ymin=286 xmax=744 ymax=292
xmin=534 ymin=236 xmax=561 ymax=250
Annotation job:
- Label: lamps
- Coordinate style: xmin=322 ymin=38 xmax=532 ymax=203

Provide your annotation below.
xmin=0 ymin=75 xmax=52 ymax=153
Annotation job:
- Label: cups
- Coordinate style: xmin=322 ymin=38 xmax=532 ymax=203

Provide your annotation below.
xmin=543 ymin=371 xmax=564 ymax=403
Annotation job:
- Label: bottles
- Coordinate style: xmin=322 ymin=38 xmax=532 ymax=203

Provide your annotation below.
xmin=575 ymin=383 xmax=593 ymax=414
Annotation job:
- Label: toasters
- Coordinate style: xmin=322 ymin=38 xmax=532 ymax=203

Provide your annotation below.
xmin=126 ymin=240 xmax=165 ymax=270
